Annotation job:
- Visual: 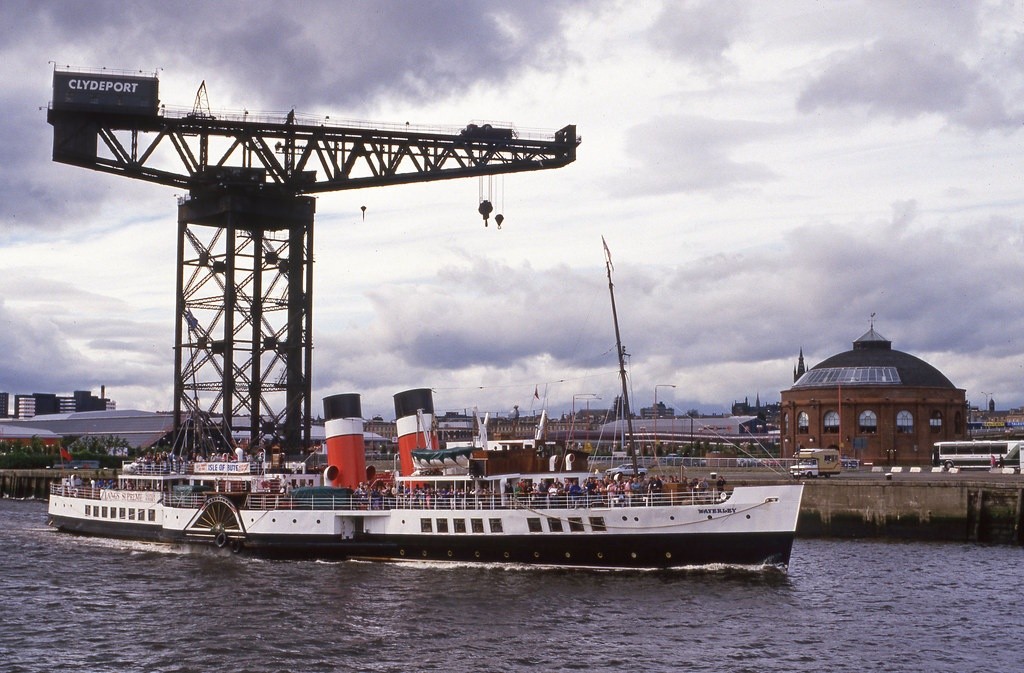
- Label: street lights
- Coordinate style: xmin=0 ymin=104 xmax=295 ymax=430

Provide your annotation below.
xmin=571 ymin=393 xmax=603 ymax=444
xmin=981 ymin=391 xmax=992 ymax=411
xmin=653 ymin=384 xmax=676 ymax=458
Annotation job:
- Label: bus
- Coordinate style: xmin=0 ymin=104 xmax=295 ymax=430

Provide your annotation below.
xmin=930 ymin=440 xmax=1024 ymax=470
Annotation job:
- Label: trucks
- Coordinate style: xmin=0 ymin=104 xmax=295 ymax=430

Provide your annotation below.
xmin=789 ymin=448 xmax=842 ymax=480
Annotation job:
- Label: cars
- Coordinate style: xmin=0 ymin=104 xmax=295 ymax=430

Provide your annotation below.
xmin=661 ymin=453 xmax=777 ymax=466
xmin=605 ymin=463 xmax=650 ymax=478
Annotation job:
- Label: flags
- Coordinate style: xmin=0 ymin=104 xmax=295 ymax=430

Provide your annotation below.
xmin=60 ymin=447 xmax=73 ymax=462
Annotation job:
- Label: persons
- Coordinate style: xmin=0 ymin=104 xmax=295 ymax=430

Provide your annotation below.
xmin=987 ymin=450 xmax=1005 ymax=471
xmin=52 ymin=450 xmax=732 ymax=509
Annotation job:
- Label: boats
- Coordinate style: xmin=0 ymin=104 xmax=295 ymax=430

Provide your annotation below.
xmin=45 ymin=387 xmax=808 ymax=582
